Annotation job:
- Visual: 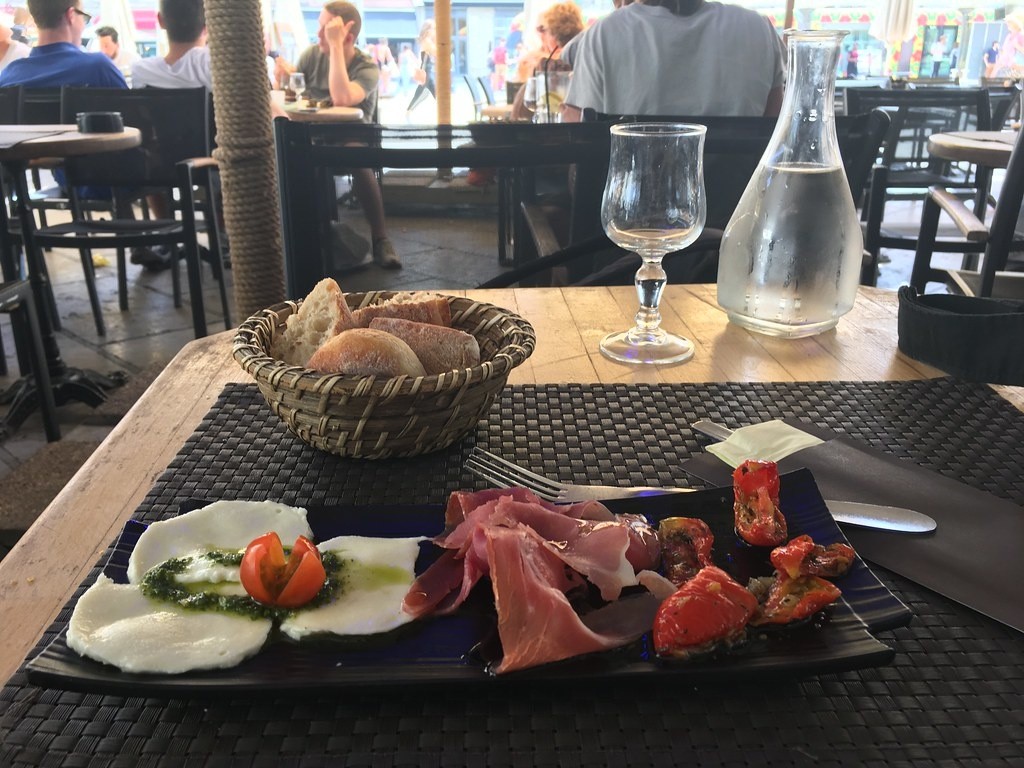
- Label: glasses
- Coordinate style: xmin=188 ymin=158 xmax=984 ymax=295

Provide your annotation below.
xmin=536 ymin=26 xmax=547 ymax=32
xmin=65 ymin=5 xmax=92 ymax=24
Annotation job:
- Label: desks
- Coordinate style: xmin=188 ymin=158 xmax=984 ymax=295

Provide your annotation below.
xmin=929 ymin=132 xmax=1018 ymax=169
xmin=0 ymin=123 xmax=145 ymax=437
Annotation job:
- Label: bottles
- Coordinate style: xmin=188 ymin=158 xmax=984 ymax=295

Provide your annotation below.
xmin=717 ymin=28 xmax=864 ymax=340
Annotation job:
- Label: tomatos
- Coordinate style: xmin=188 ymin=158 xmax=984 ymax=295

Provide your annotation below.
xmin=656 ymin=459 xmax=857 ymax=652
xmin=240 ymin=530 xmax=327 ymax=608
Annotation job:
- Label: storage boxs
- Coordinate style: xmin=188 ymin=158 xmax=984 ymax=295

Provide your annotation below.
xmin=231 ymin=290 xmax=534 ymax=461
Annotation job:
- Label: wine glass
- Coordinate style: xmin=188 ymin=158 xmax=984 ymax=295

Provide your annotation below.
xmin=599 ymin=122 xmax=707 ymax=364
xmin=289 ymin=72 xmax=306 ymax=109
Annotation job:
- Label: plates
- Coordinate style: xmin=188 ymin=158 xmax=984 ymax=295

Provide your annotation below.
xmin=22 ymin=467 xmax=913 ymax=704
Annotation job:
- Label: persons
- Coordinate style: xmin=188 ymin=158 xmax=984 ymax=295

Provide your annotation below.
xmin=950 ymin=42 xmax=959 ymax=69
xmin=0 ymin=0 xmax=403 ymax=273
xmin=929 ymin=35 xmax=947 ymax=79
xmin=360 ymin=10 xmax=525 ymax=113
xmin=983 ymin=40 xmax=1000 ymax=79
xmin=466 ymin=0 xmax=788 ymax=185
xmin=846 ymin=42 xmax=859 ymax=80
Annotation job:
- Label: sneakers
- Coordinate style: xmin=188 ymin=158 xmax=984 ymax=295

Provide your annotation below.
xmin=372 ymin=236 xmax=402 ymax=270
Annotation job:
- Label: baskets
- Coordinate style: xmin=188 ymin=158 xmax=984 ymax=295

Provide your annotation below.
xmin=233 ymin=292 xmax=536 ymax=460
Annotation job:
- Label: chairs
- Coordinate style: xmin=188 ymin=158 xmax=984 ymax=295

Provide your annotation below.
xmin=0 ymin=81 xmax=1024 ymax=440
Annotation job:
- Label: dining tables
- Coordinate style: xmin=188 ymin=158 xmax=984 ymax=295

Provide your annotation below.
xmin=275 ymin=99 xmax=363 ymax=123
xmin=0 ymin=277 xmax=1024 ymax=768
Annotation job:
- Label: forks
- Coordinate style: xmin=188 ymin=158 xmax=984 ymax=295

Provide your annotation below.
xmin=463 ymin=446 xmax=938 ymax=531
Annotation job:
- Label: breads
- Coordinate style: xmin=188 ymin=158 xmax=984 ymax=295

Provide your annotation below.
xmin=279 ymin=278 xmax=480 ymax=378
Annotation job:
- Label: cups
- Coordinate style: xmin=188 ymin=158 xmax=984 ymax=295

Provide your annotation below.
xmin=523 ymin=71 xmax=573 ymax=123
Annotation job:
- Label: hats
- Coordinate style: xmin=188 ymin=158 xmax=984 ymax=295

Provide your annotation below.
xmin=0 ymin=24 xmax=13 ymax=41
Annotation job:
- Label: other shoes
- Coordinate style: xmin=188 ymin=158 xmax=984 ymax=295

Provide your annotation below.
xmin=130 ymin=246 xmax=179 ymax=273
